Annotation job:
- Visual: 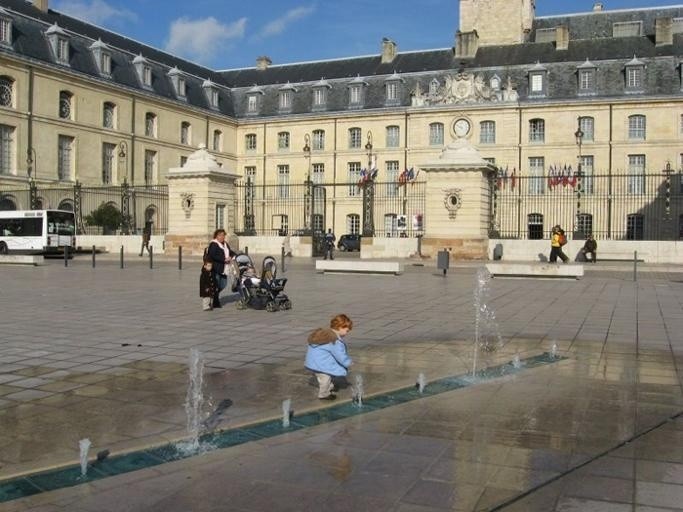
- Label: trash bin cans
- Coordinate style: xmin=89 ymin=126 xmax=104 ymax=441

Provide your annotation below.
xmin=496 ymin=244 xmax=502 ymax=260
xmin=438 ymin=251 xmax=449 ymax=274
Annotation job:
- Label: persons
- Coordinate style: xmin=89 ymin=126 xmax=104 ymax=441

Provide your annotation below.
xmin=547 ymin=225 xmax=570 ymax=263
xmin=581 ymin=235 xmax=597 ymax=263
xmin=138 ymin=222 xmax=151 ymax=256
xmin=208 ymin=229 xmax=238 ymax=309
xmin=386 ymin=233 xmax=391 ymax=237
xmin=200 ymin=258 xmax=219 ymax=311
xmin=241 ymin=267 xmax=261 ymax=287
xmin=303 ymin=314 xmax=355 ymax=401
xmin=323 ymin=228 xmax=336 ymax=260
xmin=306 ymin=419 xmax=355 ymax=482
xmin=400 ymin=233 xmax=407 ymax=237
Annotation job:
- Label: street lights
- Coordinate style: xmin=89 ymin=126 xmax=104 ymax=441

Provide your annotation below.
xmin=26 ymin=147 xmax=37 ymax=209
xmin=118 ymin=140 xmax=129 ymax=219
xmin=574 ymin=127 xmax=584 ymax=239
xmin=362 ymin=130 xmax=375 ymax=236
xmin=303 ymin=134 xmax=314 ymax=227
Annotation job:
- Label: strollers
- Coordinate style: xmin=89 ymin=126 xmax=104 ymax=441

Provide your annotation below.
xmin=227 ymin=254 xmax=291 ymax=313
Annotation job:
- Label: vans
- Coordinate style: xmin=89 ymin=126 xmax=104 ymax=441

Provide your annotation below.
xmin=337 ymin=234 xmax=363 ymax=252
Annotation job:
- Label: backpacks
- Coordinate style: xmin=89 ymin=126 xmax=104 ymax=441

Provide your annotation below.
xmin=558 ymin=234 xmax=567 ymax=245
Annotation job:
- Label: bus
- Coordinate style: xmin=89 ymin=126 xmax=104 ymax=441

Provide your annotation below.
xmin=0 ymin=210 xmax=77 ymax=258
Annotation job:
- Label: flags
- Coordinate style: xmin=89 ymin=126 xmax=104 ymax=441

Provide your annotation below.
xmin=356 ymin=169 xmax=379 ymax=188
xmin=497 ymin=165 xmax=517 ymax=192
xmin=399 ymin=166 xmax=415 ymax=185
xmin=547 ymin=165 xmax=575 ymax=190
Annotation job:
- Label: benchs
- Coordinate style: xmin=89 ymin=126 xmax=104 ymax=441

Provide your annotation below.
xmin=0 ymin=254 xmax=45 ymax=268
xmin=485 ymin=260 xmax=583 ymax=279
xmin=316 ymin=258 xmax=404 ymax=277
xmin=585 ymin=252 xmax=644 ymax=264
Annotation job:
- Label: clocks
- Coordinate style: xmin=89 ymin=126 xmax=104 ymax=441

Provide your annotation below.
xmin=450 ymin=116 xmax=472 ymax=140
